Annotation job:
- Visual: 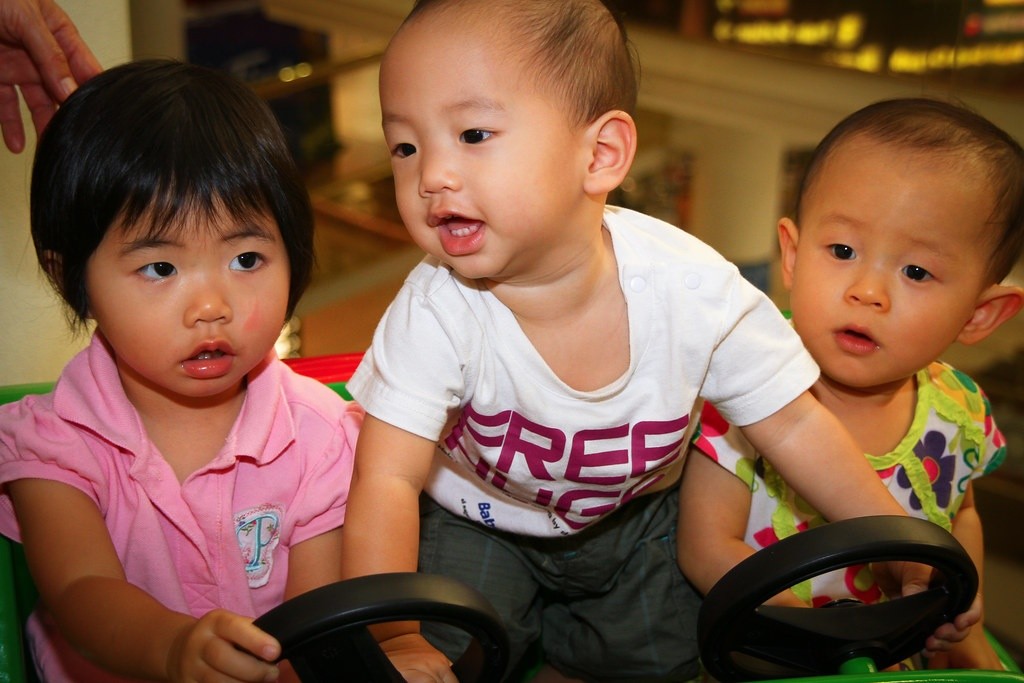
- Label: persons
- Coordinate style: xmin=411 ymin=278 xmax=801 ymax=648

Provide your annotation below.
xmin=0 ymin=0 xmax=105 ymax=154
xmin=676 ymin=97 xmax=1024 ymax=674
xmin=1 ymin=57 xmax=359 ymax=683
xmin=341 ymin=0 xmax=984 ymax=682
